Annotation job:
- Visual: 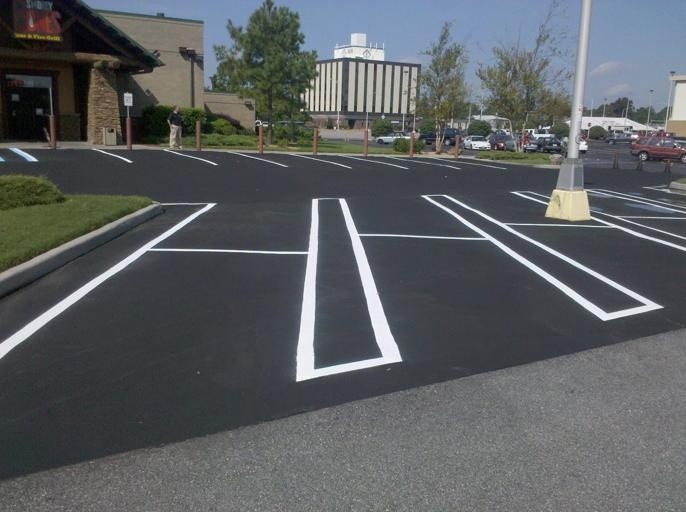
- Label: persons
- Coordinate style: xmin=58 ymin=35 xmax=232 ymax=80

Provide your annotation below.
xmin=524 ymin=130 xmax=528 ymax=146
xmin=530 ymin=130 xmax=534 ymax=142
xmin=167 ymin=105 xmax=183 ymax=151
xmin=607 ymin=125 xmax=614 ymax=138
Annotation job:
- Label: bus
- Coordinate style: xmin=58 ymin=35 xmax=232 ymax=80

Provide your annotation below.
xmin=472 ymin=115 xmax=512 ymax=138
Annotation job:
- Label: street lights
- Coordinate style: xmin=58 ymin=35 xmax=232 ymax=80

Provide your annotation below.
xmin=603 ymin=97 xmax=608 ymax=126
xmin=664 ymin=72 xmax=676 ymax=132
xmin=647 ymin=90 xmax=653 ymax=130
xmin=591 ymin=99 xmax=594 ymax=122
xmin=624 ymin=96 xmax=631 ymax=126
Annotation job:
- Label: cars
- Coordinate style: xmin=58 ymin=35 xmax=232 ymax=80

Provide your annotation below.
xmin=375 ymin=126 xmax=461 ymax=146
xmin=630 ymin=136 xmax=686 ymax=163
xmin=464 ymin=131 xmax=513 ymax=150
xmin=606 ymin=130 xmax=632 ymax=144
xmin=255 ymin=120 xmax=275 ymax=129
xmin=524 ymin=136 xmax=588 ymax=155
